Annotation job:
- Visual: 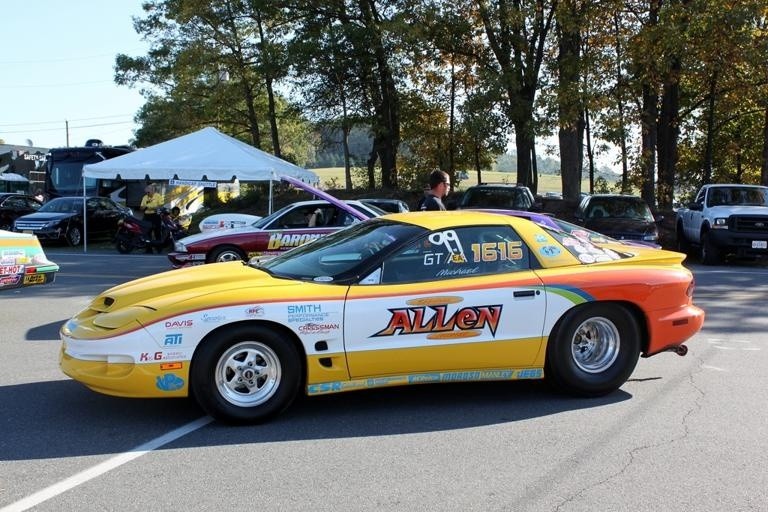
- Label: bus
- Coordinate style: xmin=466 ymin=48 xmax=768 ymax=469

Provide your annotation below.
xmin=44 ymin=139 xmax=152 ymax=207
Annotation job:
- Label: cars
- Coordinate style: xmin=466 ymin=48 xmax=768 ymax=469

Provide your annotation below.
xmin=0 ymin=230 xmax=58 ymax=291
xmin=13 ymin=196 xmax=134 ymax=248
xmin=0 ymin=194 xmax=43 ymax=230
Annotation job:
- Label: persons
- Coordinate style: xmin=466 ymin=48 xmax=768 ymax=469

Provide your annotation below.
xmin=283 ymin=210 xmax=312 ymax=229
xmin=139 ymin=185 xmax=164 ymax=257
xmin=172 ymin=206 xmax=183 ymax=233
xmin=416 ymin=183 xmax=431 ymax=211
xmin=418 ymin=170 xmax=453 ymax=212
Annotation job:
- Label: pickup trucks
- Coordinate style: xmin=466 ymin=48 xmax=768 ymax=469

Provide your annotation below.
xmin=674 ymin=183 xmax=768 ymax=266
